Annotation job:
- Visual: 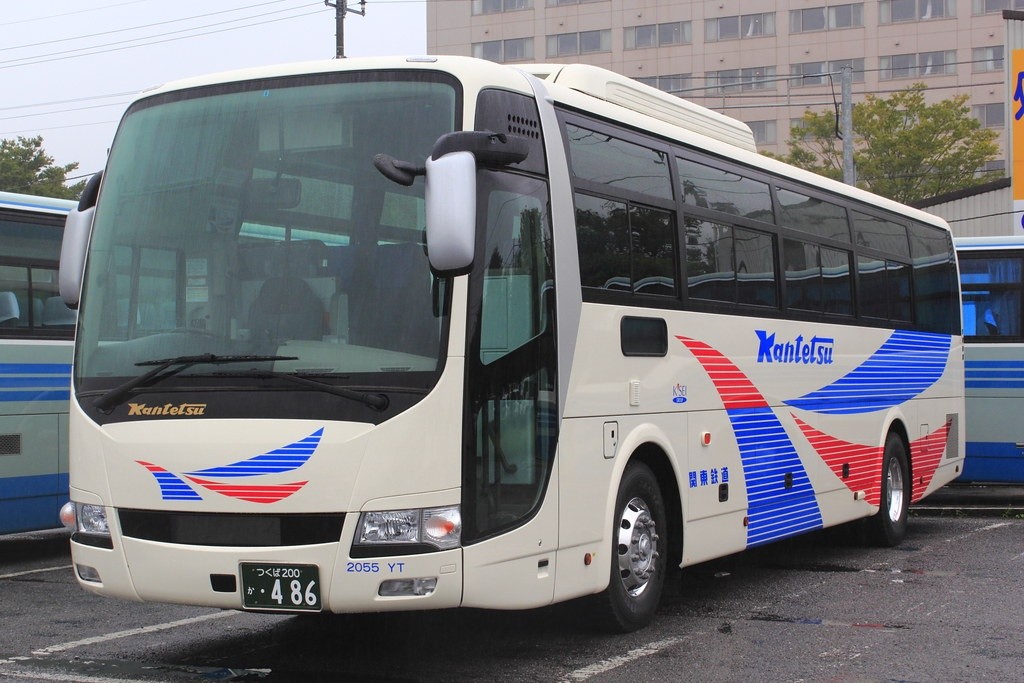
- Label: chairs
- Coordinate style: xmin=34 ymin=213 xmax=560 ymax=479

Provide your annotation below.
xmin=0 ymin=290 xmax=79 ymax=328
xmin=244 ymin=272 xmax=325 ymax=343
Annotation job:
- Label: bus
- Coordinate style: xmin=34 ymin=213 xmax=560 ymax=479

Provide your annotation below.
xmin=59 ymin=52 xmax=966 ymax=635
xmin=947 ymin=236 xmax=1024 ymax=488
xmin=1 ymin=191 xmax=430 ymax=546
